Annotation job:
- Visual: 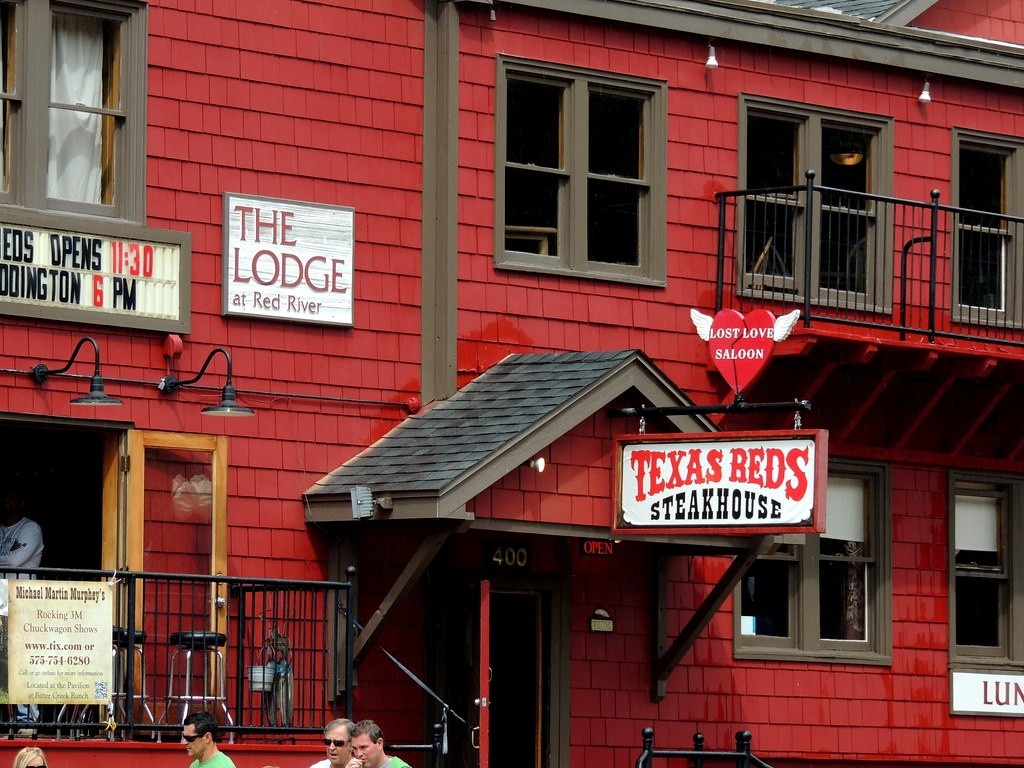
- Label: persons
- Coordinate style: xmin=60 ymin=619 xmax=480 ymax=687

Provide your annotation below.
xmin=309 ymin=719 xmax=355 ymax=768
xmin=181 ymin=712 xmax=237 ymax=768
xmin=345 ymin=720 xmax=412 ymax=768
xmin=13 ymin=747 xmax=48 ymax=768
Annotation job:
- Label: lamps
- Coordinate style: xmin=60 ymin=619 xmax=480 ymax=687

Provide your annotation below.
xmin=520 ymin=457 xmax=546 ymax=474
xmin=829 ymin=134 xmax=866 ymax=166
xmin=706 ymin=46 xmax=719 ymax=69
xmin=919 ymin=82 xmax=932 ymax=103
xmin=164 ymin=348 xmax=256 ymax=419
xmin=32 ymin=335 xmax=125 ymax=408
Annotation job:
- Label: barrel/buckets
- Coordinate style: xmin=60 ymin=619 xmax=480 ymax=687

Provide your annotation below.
xmin=247 ymin=665 xmax=275 ymax=691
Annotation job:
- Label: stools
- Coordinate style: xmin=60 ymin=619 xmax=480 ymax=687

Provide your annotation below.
xmin=56 ymin=626 xmax=156 ymax=742
xmin=0 ymin=704 xmax=41 ymax=740
xmin=157 ymin=631 xmax=234 ymax=744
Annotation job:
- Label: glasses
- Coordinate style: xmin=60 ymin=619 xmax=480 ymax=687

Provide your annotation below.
xmin=182 ymin=733 xmax=204 ymax=742
xmin=25 ymin=764 xmax=47 ymax=768
xmin=323 ymin=739 xmax=352 ymax=746
